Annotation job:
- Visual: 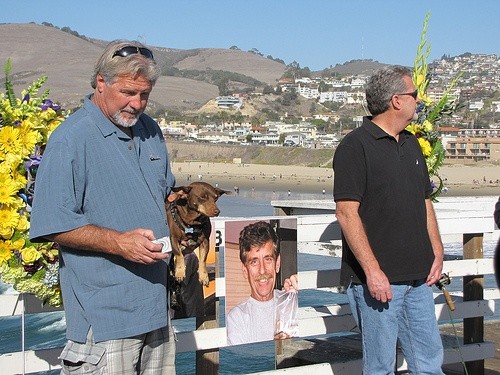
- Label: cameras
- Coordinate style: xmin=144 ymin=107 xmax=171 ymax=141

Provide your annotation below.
xmin=152 ymin=237 xmax=172 ymax=253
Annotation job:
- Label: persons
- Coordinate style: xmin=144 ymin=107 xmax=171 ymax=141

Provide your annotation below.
xmin=332 ymin=65 xmax=444 ymax=375
xmin=226 ymin=222 xmax=297 ymax=344
xmin=29 ymin=38 xmax=179 ymax=375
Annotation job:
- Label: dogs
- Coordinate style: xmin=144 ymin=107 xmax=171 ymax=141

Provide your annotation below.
xmin=163 ymin=182 xmax=235 ymax=285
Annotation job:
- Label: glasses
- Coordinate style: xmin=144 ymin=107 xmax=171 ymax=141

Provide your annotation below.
xmin=112 ymin=46 xmax=153 ymax=58
xmin=390 ymin=89 xmax=418 ymax=101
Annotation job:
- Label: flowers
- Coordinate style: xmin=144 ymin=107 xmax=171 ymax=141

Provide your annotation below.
xmin=0 ymin=57 xmax=63 ymax=308
xmin=405 ymin=8 xmax=464 ymax=202
